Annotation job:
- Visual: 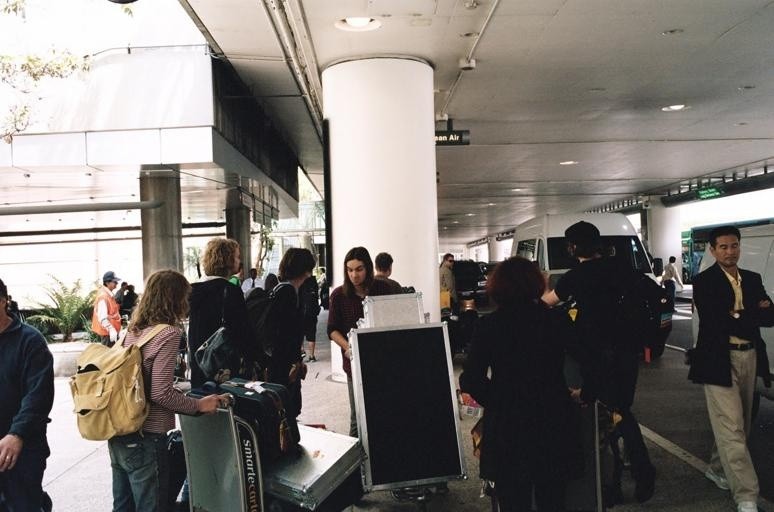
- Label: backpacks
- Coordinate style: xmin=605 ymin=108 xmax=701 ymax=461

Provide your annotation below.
xmin=70 ymin=342 xmax=151 ymax=442
xmin=301 ymin=283 xmax=320 ymax=320
xmin=242 ymin=286 xmax=272 ymax=348
xmin=614 ymin=269 xmax=660 ymax=348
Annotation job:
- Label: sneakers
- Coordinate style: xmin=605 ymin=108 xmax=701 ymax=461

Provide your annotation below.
xmin=300 ymin=351 xmax=316 ymax=362
xmin=703 ymin=466 xmax=732 ymax=490
xmin=732 ymin=493 xmax=759 ymax=512
xmin=632 ymin=465 xmax=655 ymax=505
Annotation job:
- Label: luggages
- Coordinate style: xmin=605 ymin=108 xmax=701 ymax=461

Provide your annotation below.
xmin=163 ymin=424 xmax=187 ymax=509
xmin=220 ymin=374 xmax=300 ymax=457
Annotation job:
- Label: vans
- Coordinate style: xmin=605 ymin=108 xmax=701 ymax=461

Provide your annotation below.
xmin=507 ymin=211 xmax=667 ymax=307
xmin=685 ymin=224 xmax=773 ymax=388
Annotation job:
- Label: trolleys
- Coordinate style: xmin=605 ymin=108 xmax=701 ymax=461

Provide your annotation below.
xmin=173 ymin=387 xmax=264 ymax=511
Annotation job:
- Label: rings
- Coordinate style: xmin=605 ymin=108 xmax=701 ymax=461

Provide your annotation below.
xmin=6 ymin=456 xmax=10 ymax=460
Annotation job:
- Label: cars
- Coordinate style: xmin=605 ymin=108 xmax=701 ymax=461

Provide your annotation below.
xmin=476 ymin=261 xmax=487 ymax=274
xmin=454 ymin=261 xmax=490 ymax=305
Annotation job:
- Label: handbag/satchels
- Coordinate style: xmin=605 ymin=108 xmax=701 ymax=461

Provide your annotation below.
xmin=193 ymin=328 xmax=241 ymax=384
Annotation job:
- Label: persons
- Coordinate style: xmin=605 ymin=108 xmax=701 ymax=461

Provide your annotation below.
xmin=8 ymin=295 xmax=20 ymax=317
xmin=327 ymin=244 xmax=416 ymax=437
xmin=0 ymin=279 xmax=54 ymax=512
xmin=458 ymin=255 xmax=607 ymax=511
xmin=439 ymin=256 xmax=457 ymax=305
xmin=88 ymin=240 xmax=329 ymax=511
xmin=541 ymin=221 xmax=658 ymax=508
xmin=688 ymin=225 xmax=774 ymax=512
xmin=660 ymin=256 xmax=683 ymax=312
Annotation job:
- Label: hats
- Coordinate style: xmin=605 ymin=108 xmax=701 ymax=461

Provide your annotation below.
xmin=102 ymin=271 xmax=118 ymax=283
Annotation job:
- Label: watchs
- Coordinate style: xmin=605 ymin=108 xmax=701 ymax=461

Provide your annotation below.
xmin=733 ymin=313 xmax=740 ymax=319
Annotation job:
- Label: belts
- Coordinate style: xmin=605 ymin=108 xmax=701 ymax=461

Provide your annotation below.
xmin=729 ymin=342 xmax=756 ymax=351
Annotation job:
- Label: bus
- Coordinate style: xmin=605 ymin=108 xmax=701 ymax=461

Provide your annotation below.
xmin=680 ymin=231 xmax=707 ymax=285
